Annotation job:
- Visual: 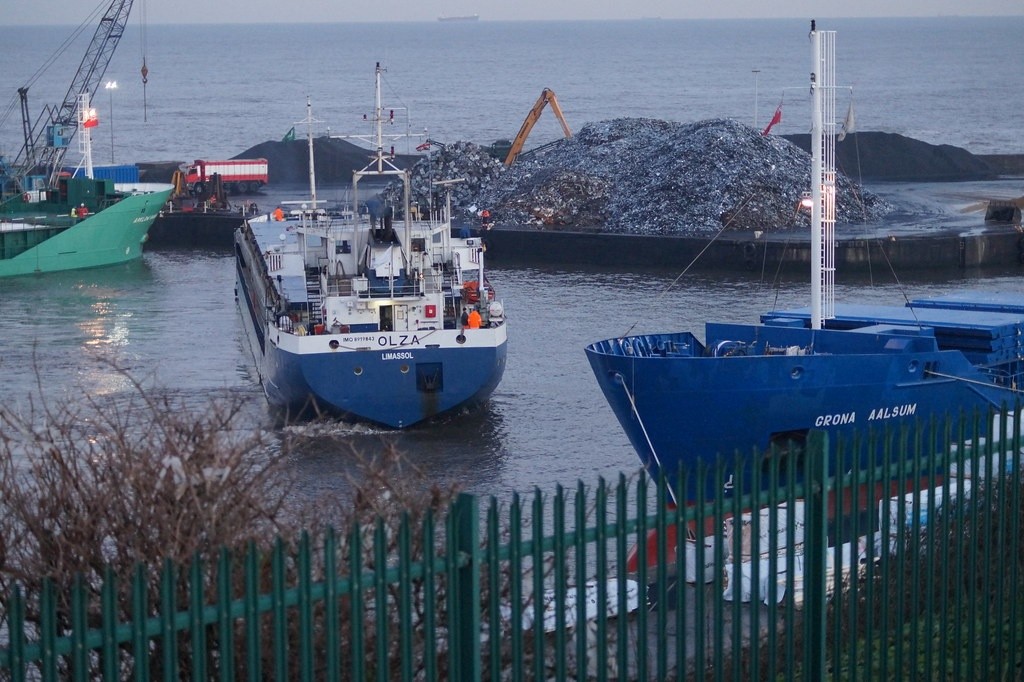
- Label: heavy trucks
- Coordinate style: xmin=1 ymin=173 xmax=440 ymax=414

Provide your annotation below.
xmin=182 ymin=158 xmax=269 ymax=194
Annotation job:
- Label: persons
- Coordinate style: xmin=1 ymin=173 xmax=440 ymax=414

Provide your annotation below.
xmin=76 ymin=203 xmax=88 ymax=224
xmin=382 ymin=312 xmax=393 ymax=332
xmin=468 ymin=308 xmax=481 ymax=329
xmin=482 ymin=208 xmax=489 ymax=228
xmin=273 ymin=206 xmax=283 ymax=221
xmin=461 ymin=307 xmax=470 ymax=329
xmin=308 ymin=318 xmax=318 ymax=335
xmin=71 ymin=205 xmax=78 ymax=225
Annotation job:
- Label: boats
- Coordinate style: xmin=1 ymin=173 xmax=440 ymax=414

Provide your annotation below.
xmin=0 ymin=85 xmax=175 ymax=278
xmin=582 ymin=27 xmax=1024 ymax=610
xmin=231 ymin=59 xmax=508 ymax=430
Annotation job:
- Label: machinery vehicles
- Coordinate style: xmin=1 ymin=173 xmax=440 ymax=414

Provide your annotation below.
xmin=504 ymin=86 xmax=573 ymax=166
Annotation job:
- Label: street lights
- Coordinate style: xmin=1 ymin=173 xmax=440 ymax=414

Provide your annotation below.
xmin=106 ymin=80 xmax=118 ymax=163
